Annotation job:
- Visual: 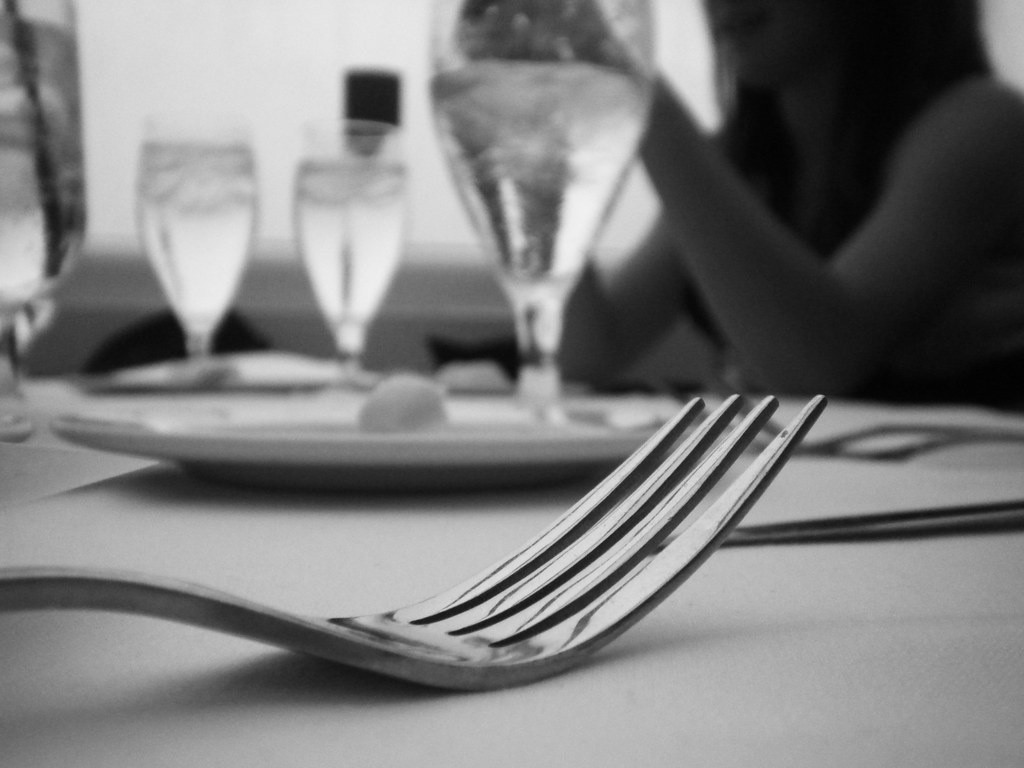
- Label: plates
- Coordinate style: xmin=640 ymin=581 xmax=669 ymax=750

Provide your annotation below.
xmin=58 ymin=379 xmax=685 ymax=494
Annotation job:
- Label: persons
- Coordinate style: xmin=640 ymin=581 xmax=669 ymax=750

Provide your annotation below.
xmin=465 ymin=0 xmax=1022 ymax=413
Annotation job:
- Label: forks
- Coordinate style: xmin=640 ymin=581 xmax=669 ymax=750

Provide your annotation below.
xmin=1 ymin=393 xmax=835 ymax=693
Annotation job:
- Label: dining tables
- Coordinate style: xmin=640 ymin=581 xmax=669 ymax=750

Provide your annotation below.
xmin=1 ymin=350 xmax=1022 ymax=767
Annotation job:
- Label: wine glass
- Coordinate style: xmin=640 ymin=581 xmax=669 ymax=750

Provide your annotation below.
xmin=427 ymin=0 xmax=653 ymax=399
xmin=1 ymin=0 xmax=88 ymax=442
xmin=291 ymin=152 xmax=412 ymax=373
xmin=139 ymin=142 xmax=260 ymax=364
